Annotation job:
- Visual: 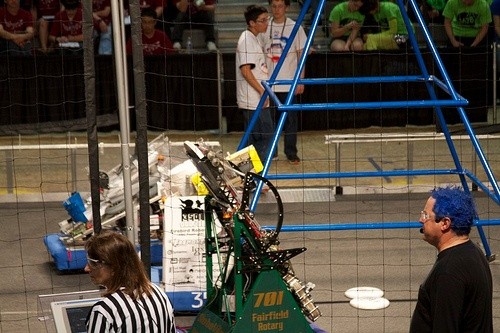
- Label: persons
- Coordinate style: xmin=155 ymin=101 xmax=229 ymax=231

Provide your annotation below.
xmin=326 ymin=0 xmax=500 ymax=52
xmin=236 ymin=4 xmax=279 ymax=162
xmin=256 ymin=0 xmax=308 ymax=163
xmin=83 ymin=229 xmax=176 ymax=333
xmin=0 ymin=0 xmax=216 ymax=58
xmin=409 ymin=184 xmax=493 ymax=333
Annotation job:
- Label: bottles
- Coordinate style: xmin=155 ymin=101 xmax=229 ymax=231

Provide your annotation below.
xmin=186 ymin=37 xmax=192 ymax=53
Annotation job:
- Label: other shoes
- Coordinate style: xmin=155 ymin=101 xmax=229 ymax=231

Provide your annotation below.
xmin=173 ymin=42 xmax=181 ymax=49
xmin=271 ymin=156 xmax=279 ymax=161
xmin=208 ymin=41 xmax=217 ymax=51
xmin=286 ymin=155 xmax=300 ymax=164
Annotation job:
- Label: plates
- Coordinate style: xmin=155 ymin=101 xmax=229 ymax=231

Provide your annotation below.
xmin=344 ymin=287 xmax=384 ymax=298
xmin=348 ymin=297 xmax=390 ymax=310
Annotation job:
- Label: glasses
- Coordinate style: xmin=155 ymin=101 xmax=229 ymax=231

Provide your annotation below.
xmin=254 ymin=16 xmax=271 ymax=23
xmin=85 ymin=252 xmax=106 ymax=270
xmin=417 ymin=211 xmax=435 ymax=223
